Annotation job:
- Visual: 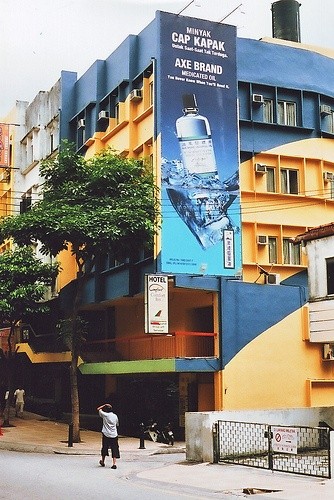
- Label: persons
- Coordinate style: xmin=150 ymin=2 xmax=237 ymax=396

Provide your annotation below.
xmin=14 ymin=385 xmax=25 ymax=418
xmin=97 ymin=404 xmax=120 ymax=469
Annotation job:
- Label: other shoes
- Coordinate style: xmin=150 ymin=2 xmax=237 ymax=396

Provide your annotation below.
xmin=110 ymin=465 xmax=117 ymax=469
xmin=99 ymin=460 xmax=106 ymax=468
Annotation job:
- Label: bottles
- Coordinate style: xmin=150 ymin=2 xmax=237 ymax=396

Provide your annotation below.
xmin=176 ymin=94 xmax=218 ymax=181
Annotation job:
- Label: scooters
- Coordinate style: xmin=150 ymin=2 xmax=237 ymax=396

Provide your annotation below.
xmin=143 ymin=422 xmax=175 ymax=446
